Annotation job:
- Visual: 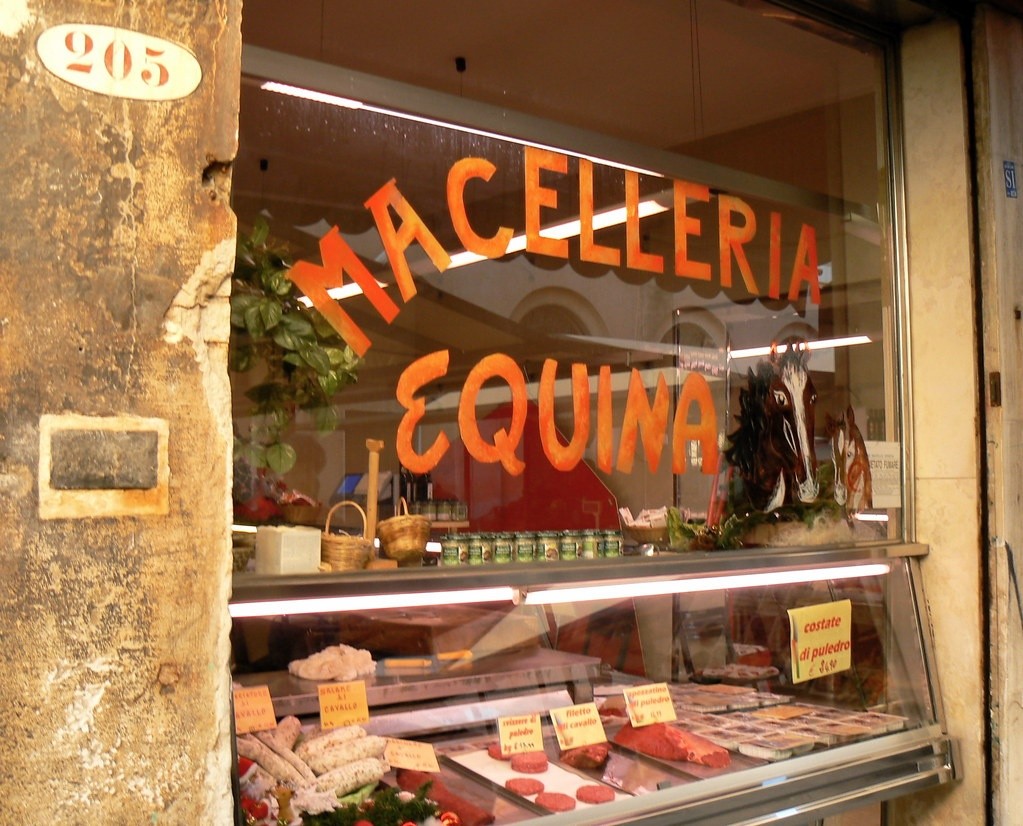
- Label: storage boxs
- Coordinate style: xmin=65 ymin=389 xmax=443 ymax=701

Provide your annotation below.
xmin=255 ymin=527 xmax=323 ymax=573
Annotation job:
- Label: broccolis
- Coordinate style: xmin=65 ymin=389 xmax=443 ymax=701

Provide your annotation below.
xmin=300 ymin=779 xmax=438 ymax=826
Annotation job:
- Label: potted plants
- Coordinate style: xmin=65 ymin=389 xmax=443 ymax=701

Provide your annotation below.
xmin=228 ymin=218 xmax=359 ymax=526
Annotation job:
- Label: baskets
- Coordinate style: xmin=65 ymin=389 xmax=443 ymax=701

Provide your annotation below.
xmin=232 ymin=537 xmax=254 ymax=573
xmin=277 ymin=499 xmax=323 ymax=525
xmin=726 ymin=511 xmax=878 ymax=547
xmin=322 ymin=500 xmax=373 ymax=574
xmin=377 ymin=497 xmax=432 ymax=562
xmin=624 ymin=522 xmax=670 ymax=543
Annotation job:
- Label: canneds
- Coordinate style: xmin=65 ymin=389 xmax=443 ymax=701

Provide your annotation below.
xmin=442 ymin=529 xmax=622 ymax=566
xmin=407 ymin=500 xmax=468 ymax=521
